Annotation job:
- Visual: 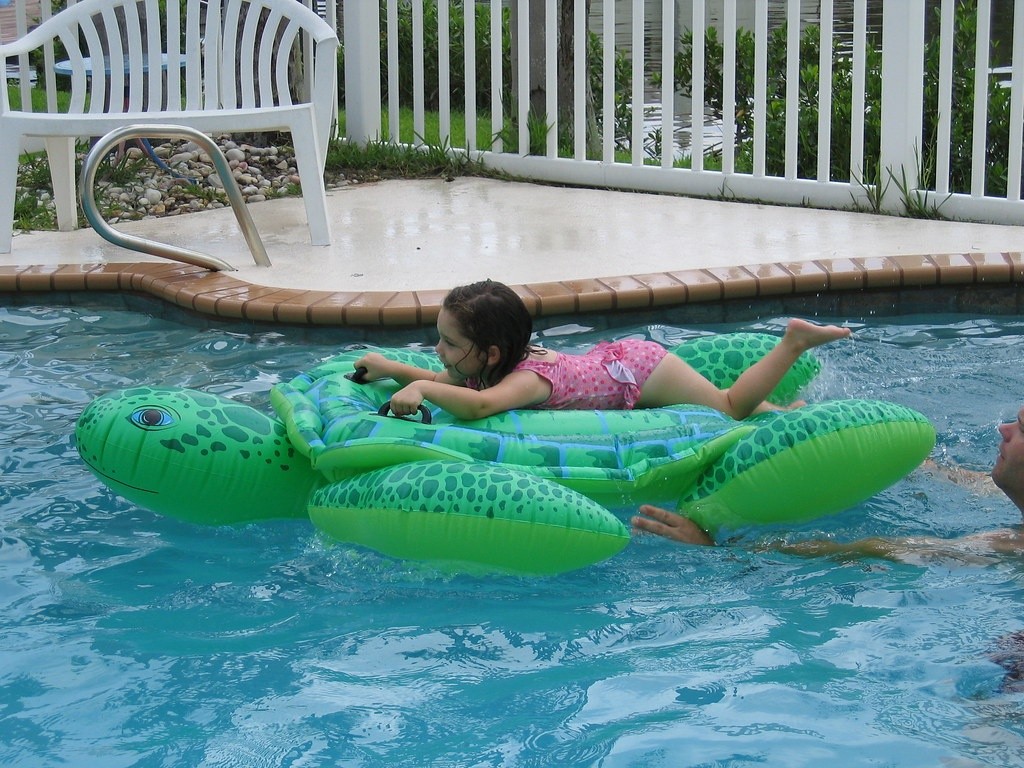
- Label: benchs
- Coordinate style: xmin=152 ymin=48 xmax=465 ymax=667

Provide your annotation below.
xmin=0 ymin=0 xmax=340 ymax=255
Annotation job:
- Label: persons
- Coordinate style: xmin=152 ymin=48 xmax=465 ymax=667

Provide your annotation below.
xmin=631 ymin=404 xmax=1024 ymax=546
xmin=352 ymin=281 xmax=851 ymax=421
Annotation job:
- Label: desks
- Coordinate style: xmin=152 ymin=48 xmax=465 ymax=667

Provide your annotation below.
xmin=54 ymin=54 xmax=200 ymax=186
